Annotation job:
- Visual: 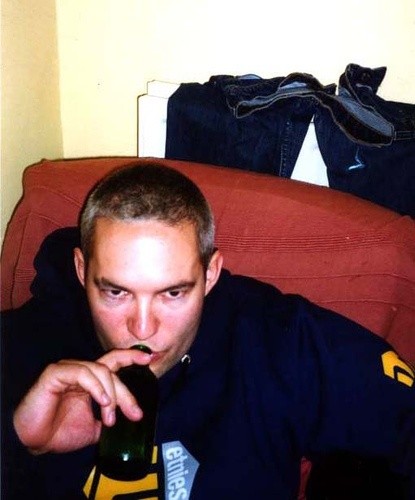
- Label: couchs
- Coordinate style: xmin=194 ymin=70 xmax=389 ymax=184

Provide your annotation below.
xmin=0 ymin=156 xmax=415 ymax=375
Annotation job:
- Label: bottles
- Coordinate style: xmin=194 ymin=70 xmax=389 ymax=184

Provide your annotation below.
xmin=95 ymin=344 xmax=162 ymax=482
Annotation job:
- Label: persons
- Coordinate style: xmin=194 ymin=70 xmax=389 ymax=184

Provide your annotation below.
xmin=0 ymin=161 xmax=415 ymax=500
